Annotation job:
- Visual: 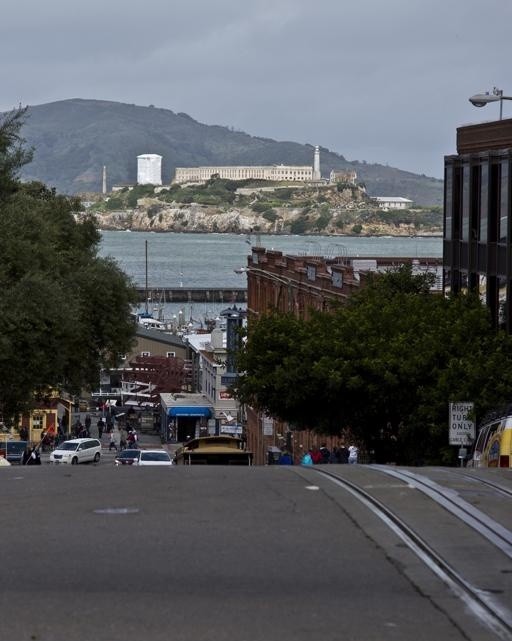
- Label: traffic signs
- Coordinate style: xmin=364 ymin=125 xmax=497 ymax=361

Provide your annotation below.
xmin=449 ymin=401 xmax=475 ymax=444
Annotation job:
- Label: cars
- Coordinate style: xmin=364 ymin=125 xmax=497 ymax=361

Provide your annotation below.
xmin=0 ymin=414 xmax=177 ymax=466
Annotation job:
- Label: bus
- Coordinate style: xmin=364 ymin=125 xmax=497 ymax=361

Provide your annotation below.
xmin=173 ymin=430 xmax=255 ymax=465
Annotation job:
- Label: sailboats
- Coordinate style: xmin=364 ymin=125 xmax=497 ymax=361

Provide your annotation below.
xmin=129 ymin=286 xmax=251 ymax=336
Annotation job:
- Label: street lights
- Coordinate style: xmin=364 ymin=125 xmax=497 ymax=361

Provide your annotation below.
xmin=469 ymin=92 xmax=512 ymax=107
xmin=233 ymin=266 xmax=293 ymax=313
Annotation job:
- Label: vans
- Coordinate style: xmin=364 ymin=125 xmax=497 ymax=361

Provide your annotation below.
xmin=472 ymin=412 xmax=512 ymax=469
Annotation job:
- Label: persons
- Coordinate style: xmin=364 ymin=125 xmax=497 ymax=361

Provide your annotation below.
xmin=30 ymin=406 xmax=140 ymax=467
xmin=278 ymin=439 xmax=361 ymax=467
